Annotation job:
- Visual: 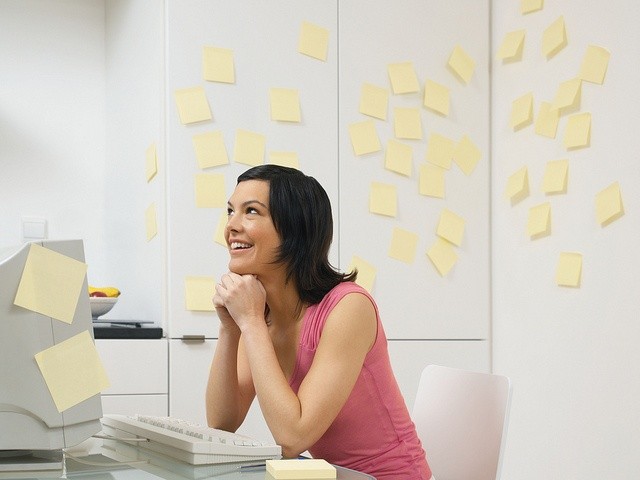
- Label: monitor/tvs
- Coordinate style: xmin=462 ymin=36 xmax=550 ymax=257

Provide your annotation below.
xmin=0 ymin=239 xmax=103 ymax=473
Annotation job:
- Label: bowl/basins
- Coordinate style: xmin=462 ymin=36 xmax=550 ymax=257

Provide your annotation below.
xmin=89 ymin=297 xmax=119 ymax=320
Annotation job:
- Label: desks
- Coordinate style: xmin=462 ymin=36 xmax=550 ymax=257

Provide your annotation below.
xmin=1 ymin=423 xmax=377 ymax=480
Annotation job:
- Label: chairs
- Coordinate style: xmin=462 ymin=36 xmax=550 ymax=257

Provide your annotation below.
xmin=410 ymin=363 xmax=513 ymax=480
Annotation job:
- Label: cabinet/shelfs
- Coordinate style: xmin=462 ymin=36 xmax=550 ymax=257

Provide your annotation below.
xmin=337 ymin=0 xmax=491 ymax=410
xmin=166 ymin=0 xmax=339 ymax=425
xmin=95 ymin=335 xmax=169 ymax=417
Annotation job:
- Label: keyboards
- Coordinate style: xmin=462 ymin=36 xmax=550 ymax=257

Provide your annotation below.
xmin=100 ymin=413 xmax=283 ymax=465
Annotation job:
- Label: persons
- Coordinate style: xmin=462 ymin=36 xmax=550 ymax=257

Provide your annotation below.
xmin=206 ymin=165 xmax=438 ymax=480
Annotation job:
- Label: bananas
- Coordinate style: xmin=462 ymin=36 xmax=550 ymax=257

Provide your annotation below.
xmin=86 ymin=285 xmax=121 ymax=298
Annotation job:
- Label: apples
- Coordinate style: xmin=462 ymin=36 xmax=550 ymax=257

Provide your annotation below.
xmin=89 ymin=291 xmax=107 ymax=298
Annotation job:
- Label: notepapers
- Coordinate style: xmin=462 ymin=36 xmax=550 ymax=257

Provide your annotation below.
xmin=265 ymin=459 xmax=337 ymax=479
